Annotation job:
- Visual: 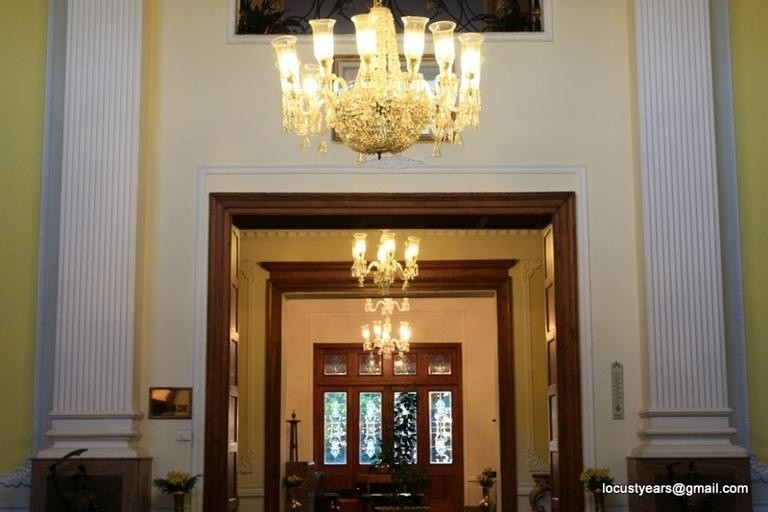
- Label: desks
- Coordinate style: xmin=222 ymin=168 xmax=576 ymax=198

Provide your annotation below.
xmin=355 ymin=473 xmax=430 ymax=512
xmin=529 ymin=473 xmax=552 ymax=512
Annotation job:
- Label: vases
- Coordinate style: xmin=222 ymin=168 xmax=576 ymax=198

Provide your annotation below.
xmin=174 ymin=494 xmax=184 ymax=512
xmin=290 ymin=488 xmax=301 ymax=512
xmin=594 ymin=493 xmax=604 ymax=512
xmin=483 ymin=488 xmax=490 ymax=512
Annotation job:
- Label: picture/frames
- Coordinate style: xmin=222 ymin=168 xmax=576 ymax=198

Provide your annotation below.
xmin=224 ymin=0 xmax=553 ymax=43
xmin=148 ymin=388 xmax=193 ymax=420
xmin=331 ymin=53 xmax=454 ymax=143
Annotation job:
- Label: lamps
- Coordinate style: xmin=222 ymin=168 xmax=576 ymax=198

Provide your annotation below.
xmin=357 ymin=296 xmax=411 ymax=352
xmin=272 ymin=4 xmax=485 ymax=161
xmin=351 ymin=229 xmax=421 ymax=291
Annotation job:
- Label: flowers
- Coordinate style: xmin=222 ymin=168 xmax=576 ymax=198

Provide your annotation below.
xmin=579 ymin=467 xmax=614 ymax=492
xmin=479 ymin=468 xmax=496 ymax=487
xmin=282 ymin=471 xmax=309 ymax=487
xmin=154 ymin=470 xmax=202 ymax=494
